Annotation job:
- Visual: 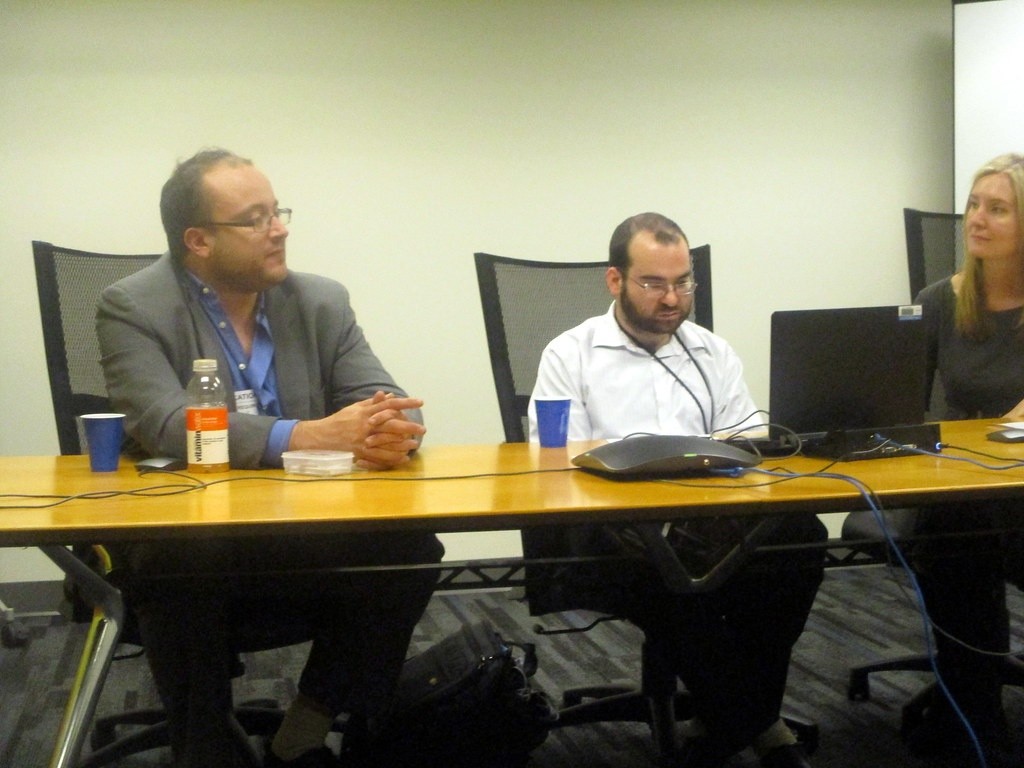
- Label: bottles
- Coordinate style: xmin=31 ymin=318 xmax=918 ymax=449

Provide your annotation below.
xmin=186 ymin=358 xmax=230 ymax=473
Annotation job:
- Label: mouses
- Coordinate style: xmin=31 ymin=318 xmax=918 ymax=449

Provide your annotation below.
xmin=135 ymin=457 xmax=186 ymax=471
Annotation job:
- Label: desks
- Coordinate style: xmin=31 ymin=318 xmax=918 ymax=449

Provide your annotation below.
xmin=1 ymin=454 xmax=1024 ymax=768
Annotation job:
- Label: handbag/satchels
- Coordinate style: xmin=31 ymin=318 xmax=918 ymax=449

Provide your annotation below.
xmin=391 ymin=622 xmax=549 ymax=767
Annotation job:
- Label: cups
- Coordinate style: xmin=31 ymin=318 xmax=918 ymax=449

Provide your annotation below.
xmin=81 ymin=412 xmax=126 ymax=472
xmin=535 ymin=399 xmax=571 ymax=448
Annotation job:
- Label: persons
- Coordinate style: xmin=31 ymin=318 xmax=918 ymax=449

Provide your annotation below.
xmin=915 ymin=153 xmax=1024 ymax=746
xmin=93 ymin=145 xmax=445 ymax=768
xmin=526 ymin=211 xmax=828 ymax=767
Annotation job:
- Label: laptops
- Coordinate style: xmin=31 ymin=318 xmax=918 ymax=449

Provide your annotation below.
xmin=727 ymin=304 xmax=932 ymax=452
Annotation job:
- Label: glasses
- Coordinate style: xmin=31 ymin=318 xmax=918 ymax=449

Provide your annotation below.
xmin=610 ymin=264 xmax=698 ymax=295
xmin=203 ymin=208 xmax=292 ymax=232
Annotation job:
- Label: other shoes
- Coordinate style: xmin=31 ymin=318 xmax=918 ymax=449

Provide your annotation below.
xmin=265 ymin=737 xmax=342 ymax=768
xmin=756 ymin=741 xmax=816 ymax=768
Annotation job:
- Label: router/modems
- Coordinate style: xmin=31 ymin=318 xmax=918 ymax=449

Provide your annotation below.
xmin=571 ymin=435 xmax=762 ymax=477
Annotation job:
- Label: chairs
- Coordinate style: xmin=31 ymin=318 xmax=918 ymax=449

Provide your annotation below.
xmin=843 ymin=206 xmax=1024 ymax=768
xmin=24 ymin=238 xmax=348 ymax=766
xmin=472 ymin=241 xmax=820 ymax=768
